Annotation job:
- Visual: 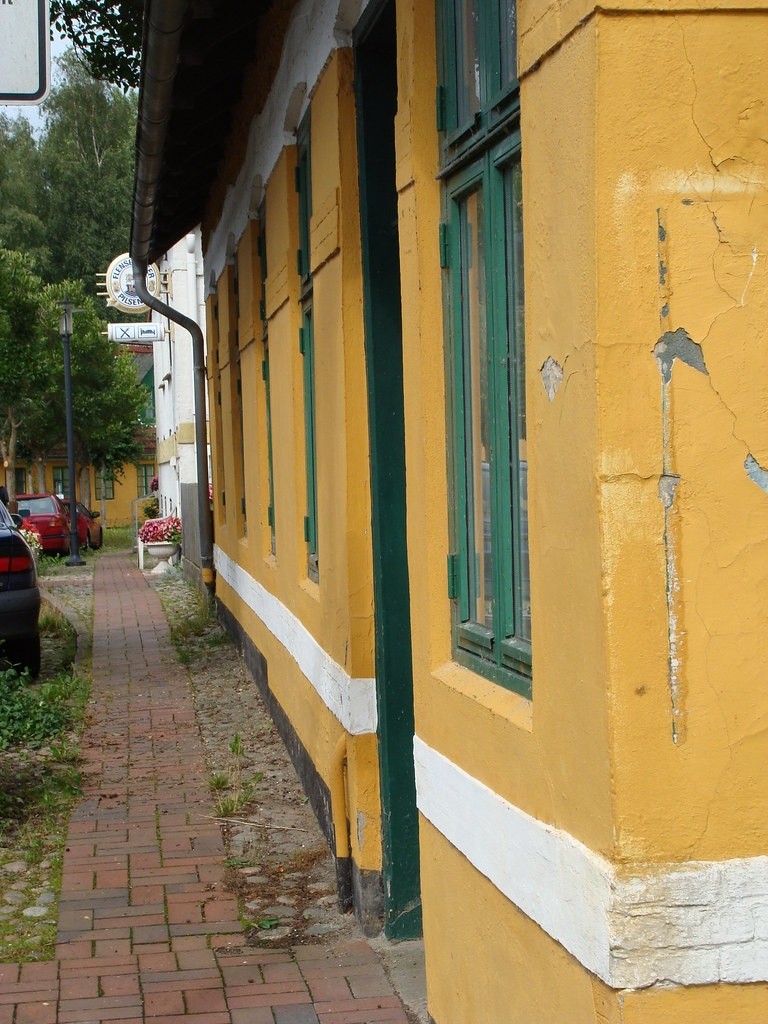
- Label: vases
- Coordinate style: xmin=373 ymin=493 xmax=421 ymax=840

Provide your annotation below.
xmin=147 ymin=541 xmax=180 ymax=574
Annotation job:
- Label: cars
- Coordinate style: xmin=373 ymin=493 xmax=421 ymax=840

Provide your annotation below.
xmin=11 ymin=490 xmax=72 ymax=558
xmin=0 ymin=499 xmax=41 ymax=681
xmin=58 ymin=496 xmax=103 ymax=550
xmin=10 ymin=508 xmax=44 ymax=559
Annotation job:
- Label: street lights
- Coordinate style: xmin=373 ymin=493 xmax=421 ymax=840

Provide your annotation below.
xmin=54 ymin=293 xmax=87 ymax=566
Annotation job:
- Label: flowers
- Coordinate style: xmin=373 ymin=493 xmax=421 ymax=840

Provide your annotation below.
xmin=138 ymin=517 xmax=183 ymax=545
